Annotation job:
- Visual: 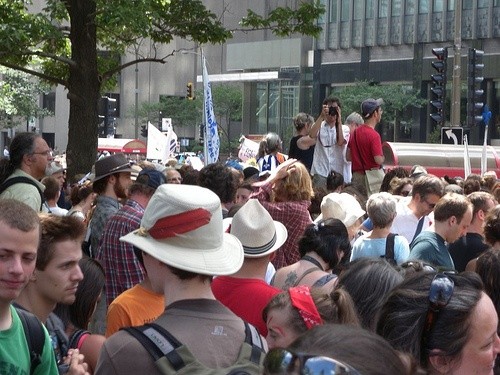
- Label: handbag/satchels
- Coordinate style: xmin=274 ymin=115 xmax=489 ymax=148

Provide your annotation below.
xmin=364 ymin=168 xmax=386 ymax=200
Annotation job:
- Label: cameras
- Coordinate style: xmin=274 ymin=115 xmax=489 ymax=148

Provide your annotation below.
xmin=328 ymin=106 xmax=338 ymax=116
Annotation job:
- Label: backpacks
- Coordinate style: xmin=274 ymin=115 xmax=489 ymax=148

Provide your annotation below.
xmin=119 ymin=322 xmax=266 ymax=375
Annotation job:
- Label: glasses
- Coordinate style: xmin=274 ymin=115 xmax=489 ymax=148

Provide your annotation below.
xmin=400 ymin=191 xmax=410 ymax=197
xmin=262 ymin=347 xmax=360 ymax=375
xmin=32 ymin=150 xmax=53 ymax=157
xmin=424 ymin=199 xmax=435 ymax=209
xmin=420 ymin=268 xmax=458 ymax=369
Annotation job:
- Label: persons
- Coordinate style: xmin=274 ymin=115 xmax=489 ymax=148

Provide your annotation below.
xmin=288 ymin=112 xmax=315 ymax=173
xmin=0 ymin=111 xmax=500 ymax=375
xmin=297 ymin=96 xmax=352 ymax=195
xmin=346 ymin=98 xmax=385 ymax=185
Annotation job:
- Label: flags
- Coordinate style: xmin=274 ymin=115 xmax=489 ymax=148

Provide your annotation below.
xmin=147 ymin=123 xmax=169 ymax=159
xmin=481 ymin=128 xmax=488 ymax=175
xmin=205 ymin=65 xmax=220 ymax=165
xmin=464 ymin=134 xmax=471 ymax=181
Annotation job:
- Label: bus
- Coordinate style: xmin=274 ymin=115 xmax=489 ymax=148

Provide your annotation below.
xmin=381 ymin=141 xmax=500 ymax=183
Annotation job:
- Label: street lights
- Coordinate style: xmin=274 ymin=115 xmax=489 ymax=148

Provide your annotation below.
xmin=181 ymin=51 xmax=214 ymax=87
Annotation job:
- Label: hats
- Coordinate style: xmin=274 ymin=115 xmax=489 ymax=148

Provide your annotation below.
xmin=361 ymin=98 xmax=383 ymax=119
xmin=47 ymin=162 xmax=70 ymax=177
xmin=134 ymin=170 xmax=167 ymax=189
xmin=119 ymin=184 xmax=244 ymax=276
xmin=87 ymin=153 xmax=139 ymax=187
xmin=408 ymin=166 xmax=428 ymax=177
xmin=314 ymin=193 xmax=366 ymax=228
xmin=223 ymin=199 xmax=287 ymax=258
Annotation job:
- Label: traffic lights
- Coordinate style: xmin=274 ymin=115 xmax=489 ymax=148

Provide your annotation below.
xmin=97 ymin=96 xmax=106 ymax=134
xmin=429 ymin=47 xmax=448 ymax=125
xmin=105 ymin=95 xmax=117 ymax=138
xmin=466 ymin=46 xmax=486 ymax=128
xmin=186 ymin=82 xmax=194 ymax=100
